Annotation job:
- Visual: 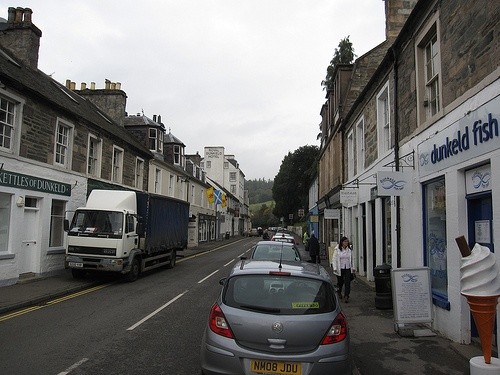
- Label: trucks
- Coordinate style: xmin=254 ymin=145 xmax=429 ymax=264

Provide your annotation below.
xmin=62 ymin=188 xmax=190 ymax=283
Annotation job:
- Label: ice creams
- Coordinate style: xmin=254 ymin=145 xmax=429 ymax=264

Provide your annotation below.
xmin=455 ymin=234 xmax=500 ymax=364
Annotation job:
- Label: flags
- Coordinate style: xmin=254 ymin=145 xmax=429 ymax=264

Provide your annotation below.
xmin=221 ymin=193 xmax=227 ymax=208
xmin=204 ymin=186 xmax=214 ymax=205
xmin=214 ymin=190 xmax=222 ymax=204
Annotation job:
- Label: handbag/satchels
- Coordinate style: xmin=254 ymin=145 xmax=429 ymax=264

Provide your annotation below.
xmin=317 ymin=256 xmax=320 ymax=263
xmin=351 ymin=272 xmax=356 ymax=281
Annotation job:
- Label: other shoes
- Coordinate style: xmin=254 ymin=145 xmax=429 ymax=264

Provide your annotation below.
xmin=337 ymin=294 xmax=350 ymax=303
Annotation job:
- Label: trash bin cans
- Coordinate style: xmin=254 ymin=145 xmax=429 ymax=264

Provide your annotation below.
xmin=373 ymin=264 xmax=392 ymax=310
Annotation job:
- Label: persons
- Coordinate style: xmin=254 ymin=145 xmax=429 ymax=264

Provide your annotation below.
xmin=258 ymin=228 xmax=262 ymax=237
xmin=263 ymin=231 xmax=269 ymax=241
xmin=332 ymin=237 xmax=356 ymax=303
xmin=307 ymin=233 xmax=320 ymax=264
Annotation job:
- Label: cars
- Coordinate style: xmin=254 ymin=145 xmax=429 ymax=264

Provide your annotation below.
xmin=249 ymin=229 xmax=259 ymax=237
xmin=239 ymin=240 xmax=302 ymax=262
xmin=262 ymin=226 xmax=299 ymax=247
xmin=199 ymin=259 xmax=352 ymax=375
